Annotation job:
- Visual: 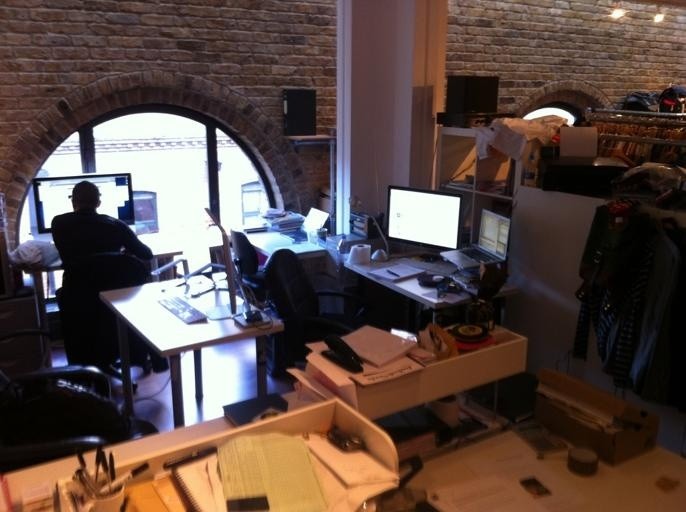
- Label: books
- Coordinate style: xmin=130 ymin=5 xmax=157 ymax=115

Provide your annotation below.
xmin=261 ymin=208 xmax=305 ymax=232
xmin=172 ymin=432 xmax=400 ymax=512
xmin=280 ymin=231 xmax=308 ymax=241
xmin=381 ymin=414 xmax=438 ymax=461
xmin=335 ymin=325 xmax=419 ymax=367
xmin=242 ymin=224 xmax=269 ymax=233
xmin=368 ymin=264 xmax=426 ymax=283
xmin=223 ymin=392 xmax=288 ymax=428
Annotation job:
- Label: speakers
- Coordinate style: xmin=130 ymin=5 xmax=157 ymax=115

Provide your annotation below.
xmin=443 ymin=76 xmax=500 ymax=128
xmin=282 ymin=86 xmax=317 ymax=137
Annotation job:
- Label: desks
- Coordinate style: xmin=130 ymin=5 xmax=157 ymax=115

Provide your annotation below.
xmin=344 ymin=246 xmax=520 ymax=425
xmin=0 ymin=397 xmax=398 ymax=512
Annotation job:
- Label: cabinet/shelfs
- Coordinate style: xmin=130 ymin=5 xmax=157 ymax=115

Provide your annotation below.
xmin=431 ymin=125 xmax=540 ymax=245
xmin=287 ymin=319 xmax=528 ymax=461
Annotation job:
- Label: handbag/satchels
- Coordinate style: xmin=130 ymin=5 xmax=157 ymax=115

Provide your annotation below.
xmin=0 ymin=374 xmax=159 ymax=443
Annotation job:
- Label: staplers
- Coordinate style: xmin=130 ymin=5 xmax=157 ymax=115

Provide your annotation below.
xmin=321 ymin=334 xmax=363 ymax=374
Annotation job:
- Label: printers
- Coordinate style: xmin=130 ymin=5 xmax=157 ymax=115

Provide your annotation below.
xmin=539 ymin=156 xmax=630 ymax=193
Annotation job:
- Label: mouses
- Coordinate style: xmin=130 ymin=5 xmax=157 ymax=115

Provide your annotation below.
xmin=432 ymin=275 xmax=444 ymax=284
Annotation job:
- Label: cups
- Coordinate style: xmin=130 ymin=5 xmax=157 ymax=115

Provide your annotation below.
xmin=79 ymin=481 xmax=125 ymax=512
xmin=316 ymin=228 xmax=328 ymax=243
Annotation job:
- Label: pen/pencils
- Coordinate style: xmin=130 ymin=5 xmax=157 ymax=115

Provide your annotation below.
xmin=163 ymin=445 xmax=217 ymax=468
xmin=387 ymin=270 xmax=400 ymax=278
xmin=70 ymin=442 xmax=151 ymax=498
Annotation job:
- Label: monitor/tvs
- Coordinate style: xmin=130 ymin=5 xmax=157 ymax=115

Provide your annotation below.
xmin=28 ymin=172 xmax=136 ymax=242
xmin=385 ymin=186 xmax=464 ymax=263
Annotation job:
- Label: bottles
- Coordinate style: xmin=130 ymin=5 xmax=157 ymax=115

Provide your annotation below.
xmin=336 ymin=235 xmax=350 ymax=262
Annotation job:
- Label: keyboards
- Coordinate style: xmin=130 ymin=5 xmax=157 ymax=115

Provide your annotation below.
xmin=158 ymin=296 xmax=209 ymax=326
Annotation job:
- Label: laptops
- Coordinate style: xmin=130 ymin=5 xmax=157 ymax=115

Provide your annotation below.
xmin=440 ymin=209 xmax=512 ymax=271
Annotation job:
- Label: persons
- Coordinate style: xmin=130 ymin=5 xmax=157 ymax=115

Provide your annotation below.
xmin=51 ymin=181 xmax=153 ymax=286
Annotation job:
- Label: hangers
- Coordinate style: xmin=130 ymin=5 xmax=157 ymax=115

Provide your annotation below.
xmin=608 ymin=195 xmax=643 ymax=223
xmin=658 ymin=203 xmax=678 ymax=229
xmin=588 ymin=111 xmax=686 ymax=163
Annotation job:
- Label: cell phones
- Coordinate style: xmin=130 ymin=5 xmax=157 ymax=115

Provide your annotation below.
xmin=227 ymin=496 xmax=269 ymax=512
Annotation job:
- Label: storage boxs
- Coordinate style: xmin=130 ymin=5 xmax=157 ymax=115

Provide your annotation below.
xmin=536 ymin=367 xmax=660 ymax=466
